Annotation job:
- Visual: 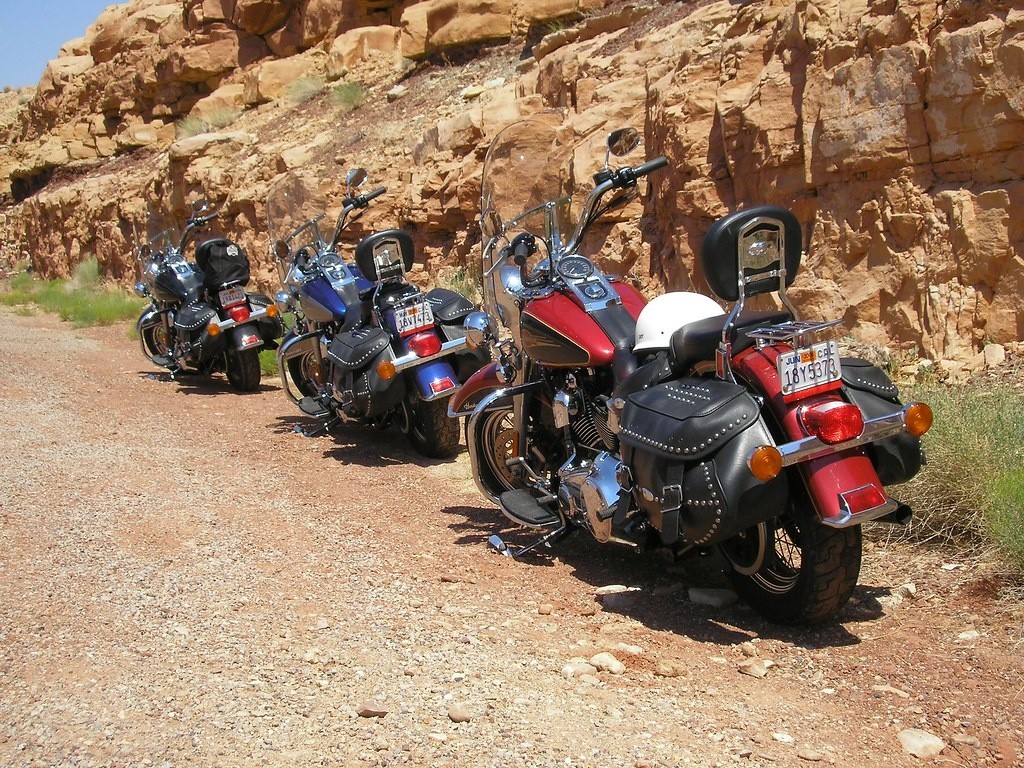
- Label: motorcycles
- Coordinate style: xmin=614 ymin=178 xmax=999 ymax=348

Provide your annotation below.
xmin=444 ymin=119 xmax=933 ymax=627
xmin=263 ymin=164 xmax=490 ymax=459
xmin=134 ymin=198 xmax=287 ymax=392
